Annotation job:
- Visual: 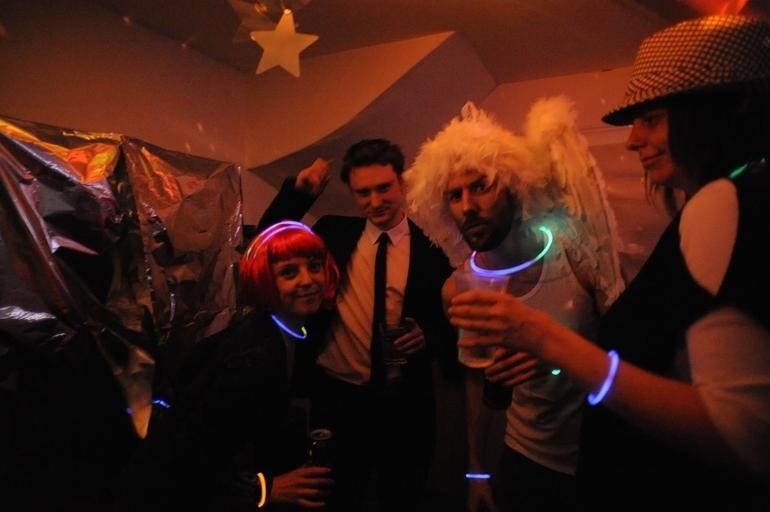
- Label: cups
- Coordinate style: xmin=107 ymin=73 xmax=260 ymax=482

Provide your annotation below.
xmin=454 ymin=271 xmax=510 ymax=366
xmin=379 ymin=318 xmax=414 ymax=366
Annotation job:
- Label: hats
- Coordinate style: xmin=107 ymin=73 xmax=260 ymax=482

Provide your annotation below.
xmin=601 ymin=14 xmax=770 ymax=125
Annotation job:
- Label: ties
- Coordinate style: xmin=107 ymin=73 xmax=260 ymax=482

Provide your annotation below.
xmin=371 ymin=233 xmax=392 ymax=383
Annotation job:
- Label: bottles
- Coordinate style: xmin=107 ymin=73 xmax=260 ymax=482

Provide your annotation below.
xmin=307 ymin=428 xmax=333 ymax=467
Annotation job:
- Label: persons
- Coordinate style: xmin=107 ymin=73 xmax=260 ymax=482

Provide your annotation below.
xmin=447 ymin=14 xmax=768 ymax=510
xmin=439 ymin=137 xmax=627 ymax=512
xmin=134 ymin=213 xmax=341 ymax=512
xmin=255 ymin=137 xmax=457 ymax=512
xmin=0 ymin=337 xmax=333 ymax=512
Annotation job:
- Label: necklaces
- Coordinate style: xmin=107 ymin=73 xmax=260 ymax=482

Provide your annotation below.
xmin=266 ymin=311 xmax=311 ymax=341
xmin=469 ymin=219 xmax=559 ymax=278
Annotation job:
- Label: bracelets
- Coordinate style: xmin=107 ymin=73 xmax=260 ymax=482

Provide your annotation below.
xmin=256 ymin=468 xmax=268 ymax=509
xmin=460 ymin=469 xmax=496 ymax=482
xmin=588 ymin=346 xmax=620 ymax=411
